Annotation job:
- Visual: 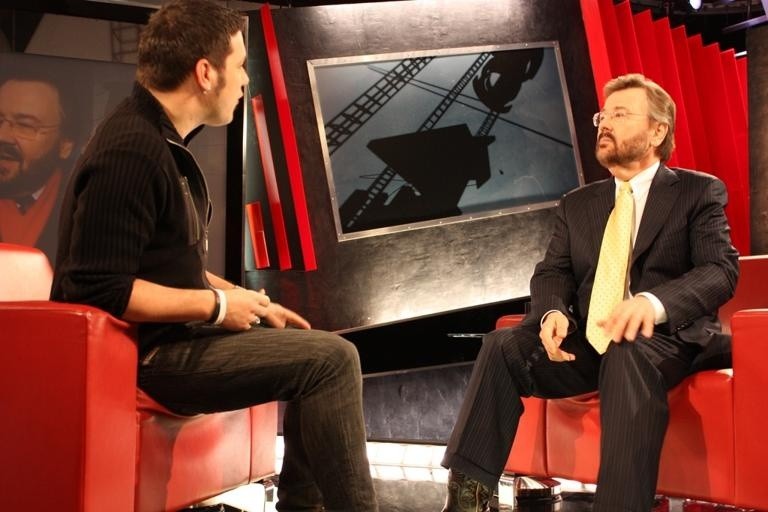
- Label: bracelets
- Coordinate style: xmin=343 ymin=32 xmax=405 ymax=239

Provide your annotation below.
xmin=213 ymin=287 xmax=226 ymax=326
xmin=206 ymin=289 xmax=221 ymax=324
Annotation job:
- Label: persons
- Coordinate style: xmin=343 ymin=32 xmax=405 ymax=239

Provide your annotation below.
xmin=441 ymin=73 xmax=742 ymax=511
xmin=2 ymin=56 xmax=89 ymax=273
xmin=49 ymin=0 xmax=380 ymax=511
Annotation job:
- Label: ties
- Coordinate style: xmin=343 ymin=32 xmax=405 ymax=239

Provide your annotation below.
xmin=585 ymin=181 xmax=635 ymax=356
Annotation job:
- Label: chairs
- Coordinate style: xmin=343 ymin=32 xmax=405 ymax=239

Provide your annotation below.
xmin=2 ymin=298 xmax=284 ymax=511
xmin=493 ymin=255 xmax=767 ymax=510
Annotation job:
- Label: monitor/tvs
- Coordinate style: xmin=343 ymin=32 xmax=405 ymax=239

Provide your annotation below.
xmin=0 ymin=0 xmax=249 ymax=303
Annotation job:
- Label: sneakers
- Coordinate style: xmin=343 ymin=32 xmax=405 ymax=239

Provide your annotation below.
xmin=440 ymin=469 xmax=495 ymax=512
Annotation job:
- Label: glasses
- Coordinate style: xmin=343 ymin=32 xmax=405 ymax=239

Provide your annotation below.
xmin=593 ymin=109 xmax=651 ymax=128
